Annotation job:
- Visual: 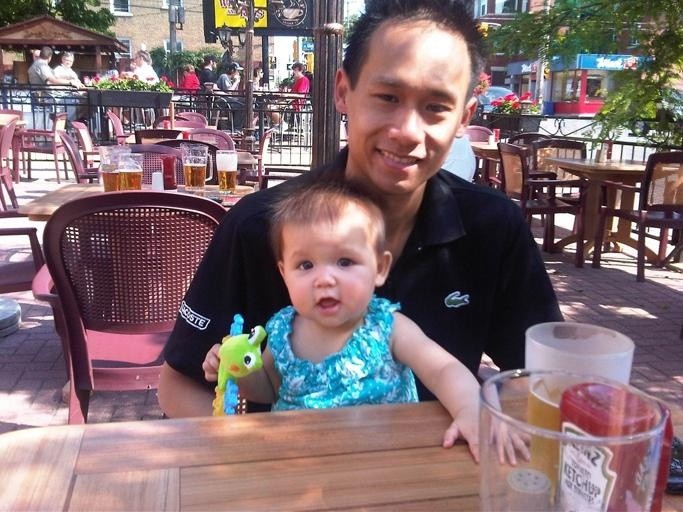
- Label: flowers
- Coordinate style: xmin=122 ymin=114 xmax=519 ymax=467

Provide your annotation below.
xmin=490 ymin=92 xmax=539 ymax=115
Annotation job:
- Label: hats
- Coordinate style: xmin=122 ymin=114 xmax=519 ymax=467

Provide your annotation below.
xmin=229 ymin=61 xmax=244 ymax=71
xmin=204 ymin=54 xmax=220 ymax=63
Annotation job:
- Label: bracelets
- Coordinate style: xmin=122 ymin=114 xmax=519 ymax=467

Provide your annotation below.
xmin=69 ymin=79 xmax=72 ymax=85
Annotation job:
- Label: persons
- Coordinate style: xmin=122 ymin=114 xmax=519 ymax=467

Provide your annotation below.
xmin=202 ymin=180 xmax=531 ymax=466
xmin=266 ymin=62 xmax=310 ymax=132
xmin=215 ymin=62 xmax=247 ymax=136
xmin=53 ymin=52 xmax=89 ymax=120
xmin=179 ymin=64 xmax=201 ymax=96
xmin=32 ymin=49 xmax=40 ymax=64
xmin=28 ymin=46 xmax=79 ymax=130
xmin=201 ymin=55 xmax=221 ymax=129
xmin=125 ymin=58 xmax=136 ymax=72
xmin=157 ymin=2 xmax=564 ymax=417
xmin=253 ymin=67 xmax=264 ymax=91
xmin=122 ymin=49 xmax=159 ymax=84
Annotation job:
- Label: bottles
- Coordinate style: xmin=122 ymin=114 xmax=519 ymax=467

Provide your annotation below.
xmin=557 ymin=382 xmax=673 ymax=511
xmin=493 ymin=128 xmax=500 ymax=142
xmin=163 ymin=121 xmax=169 ymax=128
xmin=488 ymin=133 xmax=494 ymax=145
xmin=160 ymin=154 xmax=178 ymax=189
xmin=182 ymin=132 xmax=190 ymax=140
xmin=151 ymin=171 xmax=164 ymax=192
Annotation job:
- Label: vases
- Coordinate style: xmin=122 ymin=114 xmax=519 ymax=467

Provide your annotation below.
xmin=480 ymin=112 xmax=545 ymax=136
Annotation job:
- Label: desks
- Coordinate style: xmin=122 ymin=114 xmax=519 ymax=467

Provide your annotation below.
xmin=1 ymin=377 xmax=683 ymax=510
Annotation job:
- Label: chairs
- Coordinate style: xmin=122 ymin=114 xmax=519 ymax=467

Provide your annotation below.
xmin=32 ymin=190 xmax=227 ymax=426
xmin=469 ymin=131 xmax=683 ymax=283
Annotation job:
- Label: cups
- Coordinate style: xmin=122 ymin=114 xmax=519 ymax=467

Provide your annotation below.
xmin=477 ymin=368 xmax=668 ymax=512
xmin=116 ymin=153 xmax=143 ymax=191
xmin=215 ymin=147 xmax=239 ymax=195
xmin=179 ymin=142 xmax=214 ymax=194
xmin=99 ymin=146 xmax=132 ymax=192
xmin=525 ymin=320 xmax=636 ymax=500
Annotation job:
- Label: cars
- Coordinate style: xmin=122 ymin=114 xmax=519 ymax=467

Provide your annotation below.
xmin=471 ymin=86 xmax=519 ymax=126
xmin=630 ymin=87 xmax=683 ymax=136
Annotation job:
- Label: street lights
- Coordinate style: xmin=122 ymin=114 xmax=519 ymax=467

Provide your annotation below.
xmin=214 ymin=20 xmax=247 ymax=55
